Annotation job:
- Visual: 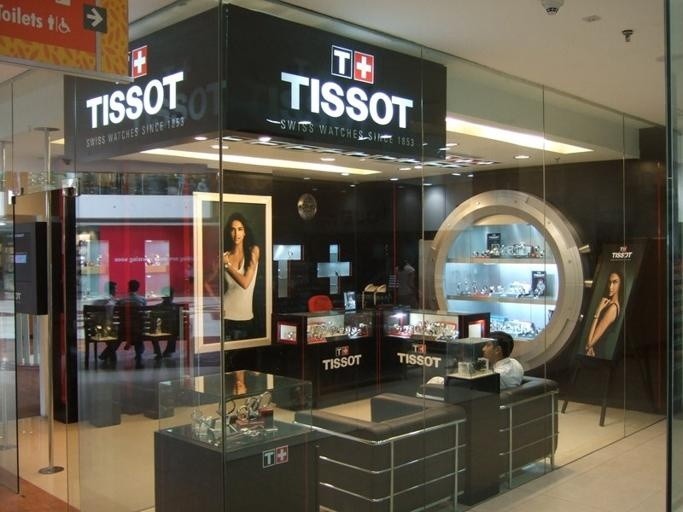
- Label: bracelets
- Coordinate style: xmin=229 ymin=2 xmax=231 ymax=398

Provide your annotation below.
xmin=391 ymin=318 xmax=455 ymax=340
xmin=593 ymin=312 xmax=599 ymax=320
xmin=471 ymin=241 xmax=544 ymax=258
xmin=456 ymin=279 xmax=544 ymax=298
xmin=490 ymin=319 xmax=538 ymax=337
xmin=288 ymin=321 xmax=361 ymax=341
xmin=188 ymin=391 xmax=272 ymax=447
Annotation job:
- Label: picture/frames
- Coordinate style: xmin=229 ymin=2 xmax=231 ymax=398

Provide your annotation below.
xmin=575 ymin=241 xmax=646 ymax=361
xmin=192 ymin=191 xmax=272 ymax=355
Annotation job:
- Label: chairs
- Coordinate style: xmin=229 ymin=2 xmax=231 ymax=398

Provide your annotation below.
xmin=292 ymin=368 xmax=559 ymax=512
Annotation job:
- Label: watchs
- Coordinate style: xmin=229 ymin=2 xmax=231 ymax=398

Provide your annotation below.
xmin=222 ymin=262 xmax=232 ymax=271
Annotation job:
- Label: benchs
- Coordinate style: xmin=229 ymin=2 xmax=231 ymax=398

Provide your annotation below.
xmin=83 ymin=304 xmax=185 ymax=371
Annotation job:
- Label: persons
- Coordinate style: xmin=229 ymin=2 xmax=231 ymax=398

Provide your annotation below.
xmin=116 ymin=280 xmax=148 ymax=369
xmin=582 ymin=270 xmax=624 ymax=358
xmin=389 ymin=256 xmax=416 ymax=303
xmin=91 ymin=280 xmax=123 ymax=368
xmin=414 ymin=331 xmax=526 ymax=403
xmin=148 ymin=286 xmax=180 ymax=360
xmin=203 ymin=212 xmax=261 ymax=398
